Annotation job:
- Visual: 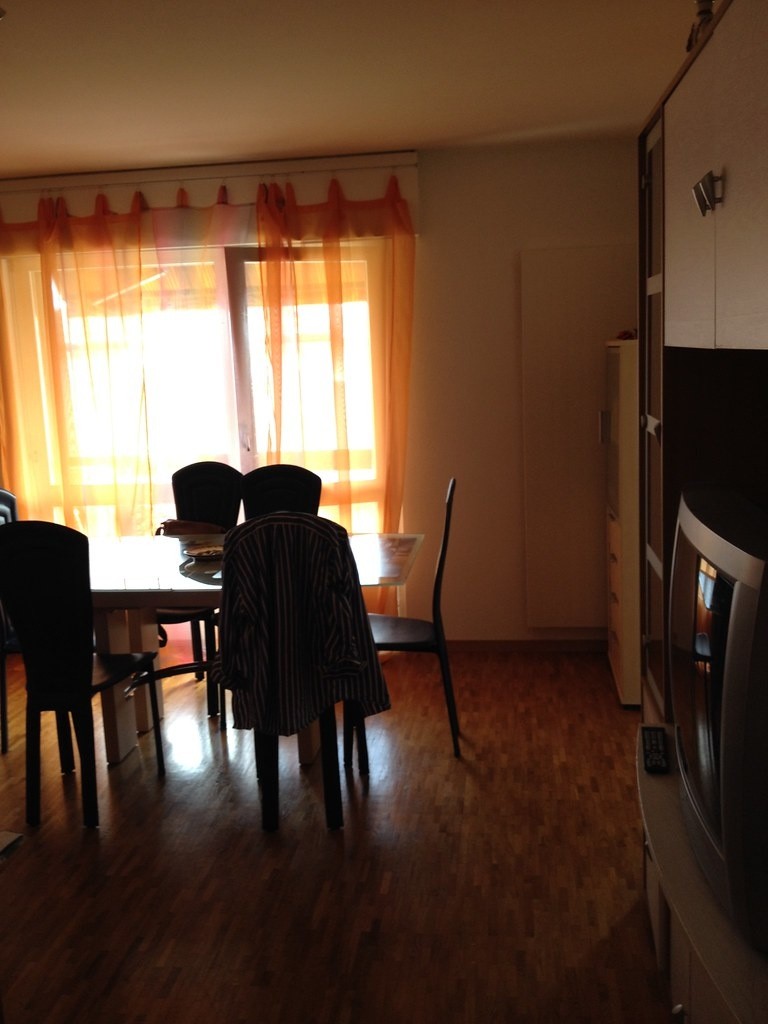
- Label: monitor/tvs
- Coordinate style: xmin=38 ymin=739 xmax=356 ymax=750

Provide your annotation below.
xmin=669 ymin=491 xmax=767 ymax=926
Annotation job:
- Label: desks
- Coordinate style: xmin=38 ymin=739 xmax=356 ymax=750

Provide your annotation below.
xmin=89 ymin=532 xmax=425 ymax=765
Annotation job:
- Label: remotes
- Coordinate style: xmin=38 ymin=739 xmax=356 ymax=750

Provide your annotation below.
xmin=641 ymin=726 xmax=669 ymax=774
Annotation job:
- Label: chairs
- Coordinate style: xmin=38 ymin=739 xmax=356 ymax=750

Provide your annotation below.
xmin=154 ymin=462 xmax=245 ymax=680
xmin=240 ymin=464 xmax=322 ymax=520
xmin=1 ymin=489 xmax=18 ymax=755
xmin=348 ymin=476 xmax=461 ymax=773
xmin=1 ymin=517 xmax=167 ymax=830
xmin=223 ymin=512 xmax=391 ymax=834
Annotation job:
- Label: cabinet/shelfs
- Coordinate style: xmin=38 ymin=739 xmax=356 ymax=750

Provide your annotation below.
xmin=602 ymin=338 xmax=641 ymax=710
xmin=633 ymin=725 xmax=768 ymax=1024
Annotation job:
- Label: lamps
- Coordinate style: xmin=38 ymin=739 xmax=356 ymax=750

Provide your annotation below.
xmin=692 ymin=170 xmax=722 ymax=217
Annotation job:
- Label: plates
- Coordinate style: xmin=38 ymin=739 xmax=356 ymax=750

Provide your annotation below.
xmin=183 ymin=543 xmax=223 ymax=559
